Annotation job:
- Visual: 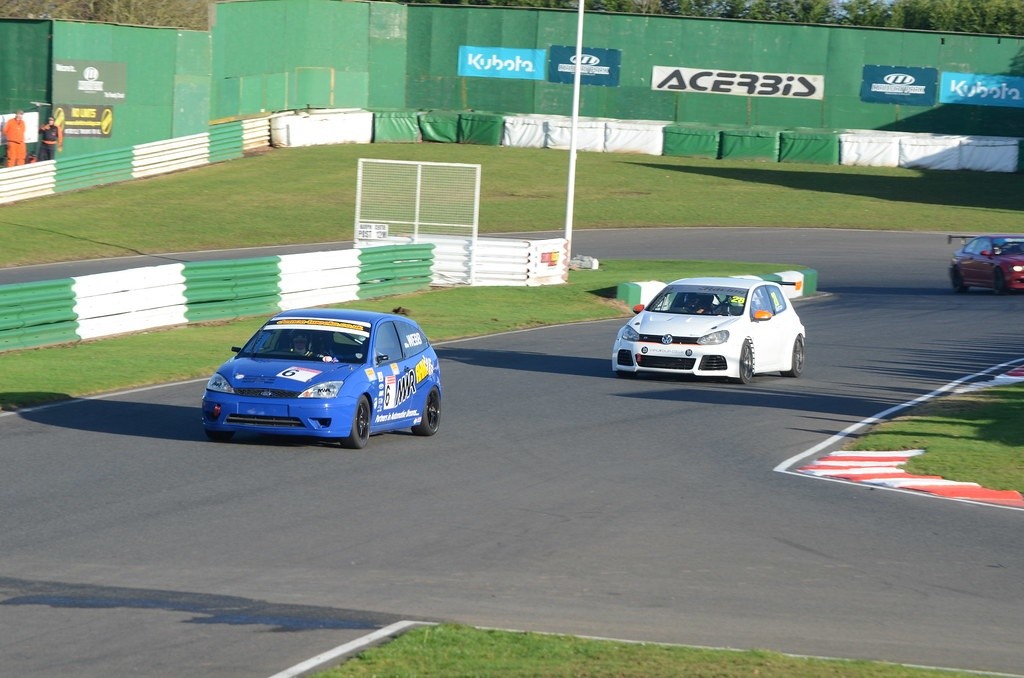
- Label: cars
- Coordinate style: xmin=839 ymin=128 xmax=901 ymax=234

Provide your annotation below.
xmin=201 ymin=307 xmax=444 ymax=451
xmin=611 ymin=277 xmax=807 ymax=386
xmin=948 ymin=233 xmax=1024 ymax=296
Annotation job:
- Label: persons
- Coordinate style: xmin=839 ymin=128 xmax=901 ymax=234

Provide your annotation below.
xmin=678 ymin=293 xmax=709 ymax=314
xmin=38 ymin=116 xmax=62 ymax=161
xmin=279 ymin=330 xmax=324 ymax=357
xmin=3 ymin=109 xmax=26 ymax=168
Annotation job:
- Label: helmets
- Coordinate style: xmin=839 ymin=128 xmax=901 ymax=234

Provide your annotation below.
xmin=289 ymin=329 xmax=311 ymax=352
